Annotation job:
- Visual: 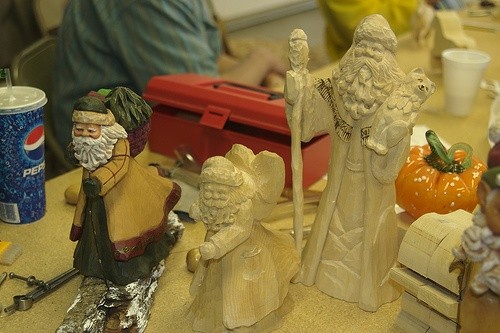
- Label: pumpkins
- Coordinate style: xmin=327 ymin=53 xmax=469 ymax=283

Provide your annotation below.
xmin=393 ymin=130 xmax=487 ymax=218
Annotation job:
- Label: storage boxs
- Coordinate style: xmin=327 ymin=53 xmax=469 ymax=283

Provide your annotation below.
xmin=143 ymin=73 xmax=330 ymax=191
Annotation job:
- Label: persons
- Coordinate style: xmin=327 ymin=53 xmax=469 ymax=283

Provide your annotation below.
xmin=188 ymin=142 xmax=301 ymax=328
xmin=451 ymin=140 xmax=500 ymax=332
xmin=50 ymin=0 xmax=291 ymax=175
xmin=318 ymin=0 xmax=431 ymax=64
xmin=69 ymin=94 xmax=182 ymax=286
xmin=285 ymin=12 xmax=413 ymax=313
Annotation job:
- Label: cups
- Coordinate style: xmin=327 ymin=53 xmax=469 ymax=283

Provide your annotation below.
xmin=441 ymin=50 xmax=491 ymax=116
xmin=0 ymin=85 xmax=48 ymax=225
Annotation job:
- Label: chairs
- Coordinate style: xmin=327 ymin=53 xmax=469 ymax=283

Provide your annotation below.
xmin=13 ymin=26 xmax=89 ymax=175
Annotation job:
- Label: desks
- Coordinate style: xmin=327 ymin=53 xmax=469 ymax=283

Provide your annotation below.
xmin=0 ymin=0 xmax=500 ymax=333
